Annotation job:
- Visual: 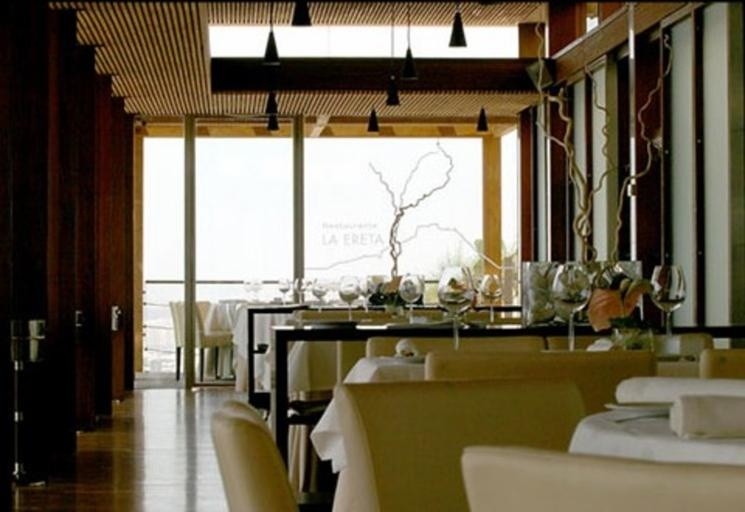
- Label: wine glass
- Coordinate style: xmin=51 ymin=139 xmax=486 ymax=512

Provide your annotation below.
xmin=552 ymin=263 xmax=591 ymax=351
xmin=294 ymin=278 xmax=307 ymax=305
xmin=339 ymin=275 xmax=358 ymax=321
xmin=479 ymin=274 xmax=504 ymax=322
xmin=252 ymin=278 xmax=264 ymax=304
xmin=313 ymin=278 xmax=326 ymax=311
xmin=278 ymin=278 xmax=289 ymax=306
xmin=650 ymin=265 xmax=688 ymax=336
xmin=243 ymin=277 xmax=252 ymax=306
xmin=399 ymin=273 xmax=421 ymax=324
xmin=437 ymin=265 xmax=474 ymax=352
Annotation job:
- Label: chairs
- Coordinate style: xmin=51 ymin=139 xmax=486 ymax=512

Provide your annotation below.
xmin=291 ymin=307 xmax=388 ymax=493
xmin=170 ymin=300 xmax=235 ymax=380
xmin=459 ymin=445 xmax=745 ymax=512
xmin=426 ymin=349 xmax=651 ymax=413
xmin=209 ymin=404 xmax=301 ymax=512
xmin=332 ymin=373 xmax=576 ymax=512
xmin=406 ymin=308 xmax=503 ymax=321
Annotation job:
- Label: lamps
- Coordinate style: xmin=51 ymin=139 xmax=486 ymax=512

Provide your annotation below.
xmin=449 ymin=2 xmax=467 ymax=48
xmin=291 ymin=1 xmax=311 ymax=27
xmin=264 ymin=2 xmax=282 ymax=67
xmin=387 ymin=2 xmax=399 ymax=106
xmin=477 ymin=107 xmax=490 ymax=132
xmin=265 ymin=85 xmax=280 ymax=117
xmin=267 ymin=115 xmax=279 ymax=131
xmin=367 ymin=110 xmax=380 ymax=132
xmin=402 ymin=2 xmax=416 ymax=79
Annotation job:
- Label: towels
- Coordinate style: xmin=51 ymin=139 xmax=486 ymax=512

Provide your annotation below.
xmin=671 ymin=394 xmax=744 ymax=438
xmin=616 ymin=375 xmax=744 ymax=403
